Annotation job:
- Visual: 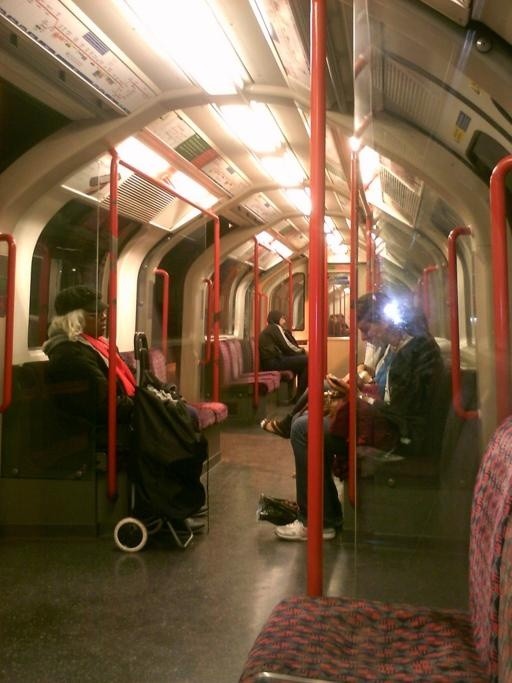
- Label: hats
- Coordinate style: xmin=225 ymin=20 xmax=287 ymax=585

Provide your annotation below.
xmin=55 ymin=284 xmax=110 ymax=316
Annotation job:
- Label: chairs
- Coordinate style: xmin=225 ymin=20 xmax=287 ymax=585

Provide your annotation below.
xmin=333 ymin=352 xmax=477 ymax=537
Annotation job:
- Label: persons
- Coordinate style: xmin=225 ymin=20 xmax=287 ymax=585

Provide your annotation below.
xmin=327 ymin=313 xmax=349 ymax=336
xmin=258 ymin=308 xmax=309 ymax=403
xmin=273 ymin=290 xmax=446 ymax=541
xmin=41 ymin=283 xmax=211 ymax=534
xmin=260 ymin=343 xmax=394 ymax=438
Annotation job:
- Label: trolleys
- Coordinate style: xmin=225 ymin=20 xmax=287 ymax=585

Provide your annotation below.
xmin=110 ymin=328 xmax=210 ymax=556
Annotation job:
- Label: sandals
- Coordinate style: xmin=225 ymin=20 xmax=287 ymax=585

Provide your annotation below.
xmin=260 ymin=419 xmax=290 ymax=439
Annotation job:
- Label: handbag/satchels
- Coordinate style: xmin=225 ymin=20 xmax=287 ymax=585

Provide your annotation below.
xmin=328 ymin=396 xmax=399 ymax=451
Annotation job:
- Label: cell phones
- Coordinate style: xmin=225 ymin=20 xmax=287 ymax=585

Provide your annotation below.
xmin=331 ymin=375 xmax=341 ymax=386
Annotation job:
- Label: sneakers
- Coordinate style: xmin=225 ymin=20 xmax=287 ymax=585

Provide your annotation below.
xmin=190 ymin=503 xmax=208 ymax=518
xmin=274 ymin=517 xmax=344 ymax=540
xmin=161 ymin=516 xmax=203 ymax=534
